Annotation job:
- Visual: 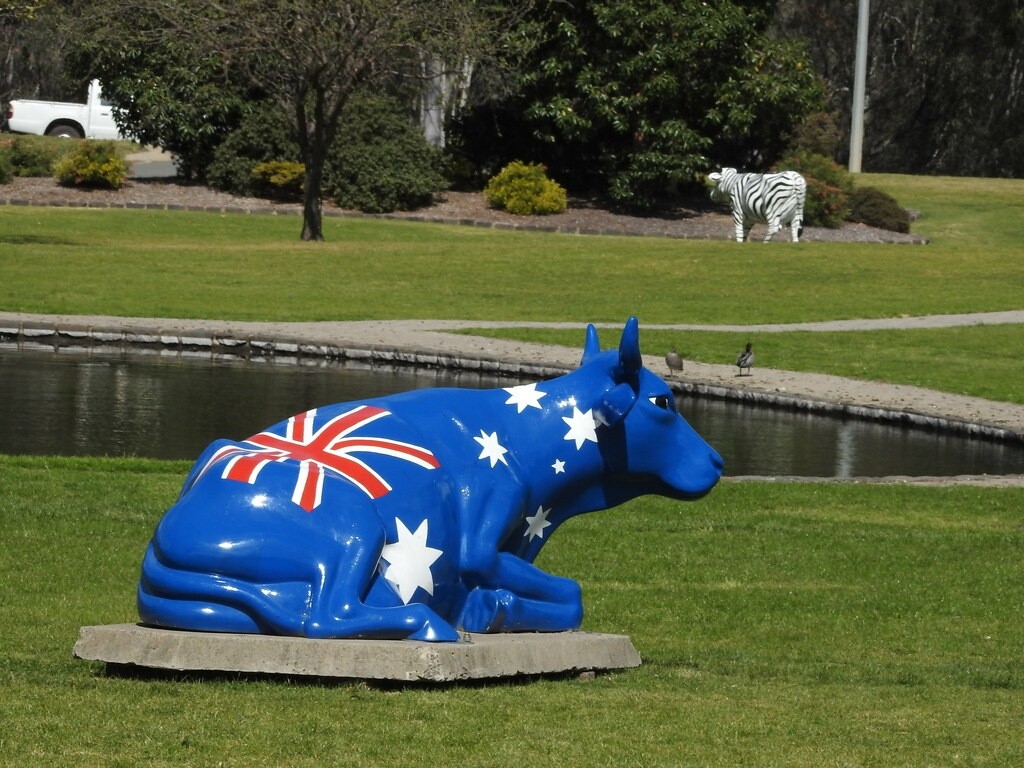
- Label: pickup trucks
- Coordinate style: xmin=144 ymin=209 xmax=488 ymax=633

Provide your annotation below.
xmin=5 ymin=78 xmax=145 ymax=144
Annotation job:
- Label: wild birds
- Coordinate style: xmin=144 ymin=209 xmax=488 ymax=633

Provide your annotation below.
xmin=665 ymin=335 xmax=754 ymax=378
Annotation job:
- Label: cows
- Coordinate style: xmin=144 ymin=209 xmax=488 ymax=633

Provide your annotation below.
xmin=133 ymin=318 xmax=725 ymax=642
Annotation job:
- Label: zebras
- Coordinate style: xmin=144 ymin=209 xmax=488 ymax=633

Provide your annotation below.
xmin=709 ymin=166 xmax=807 ymax=244
